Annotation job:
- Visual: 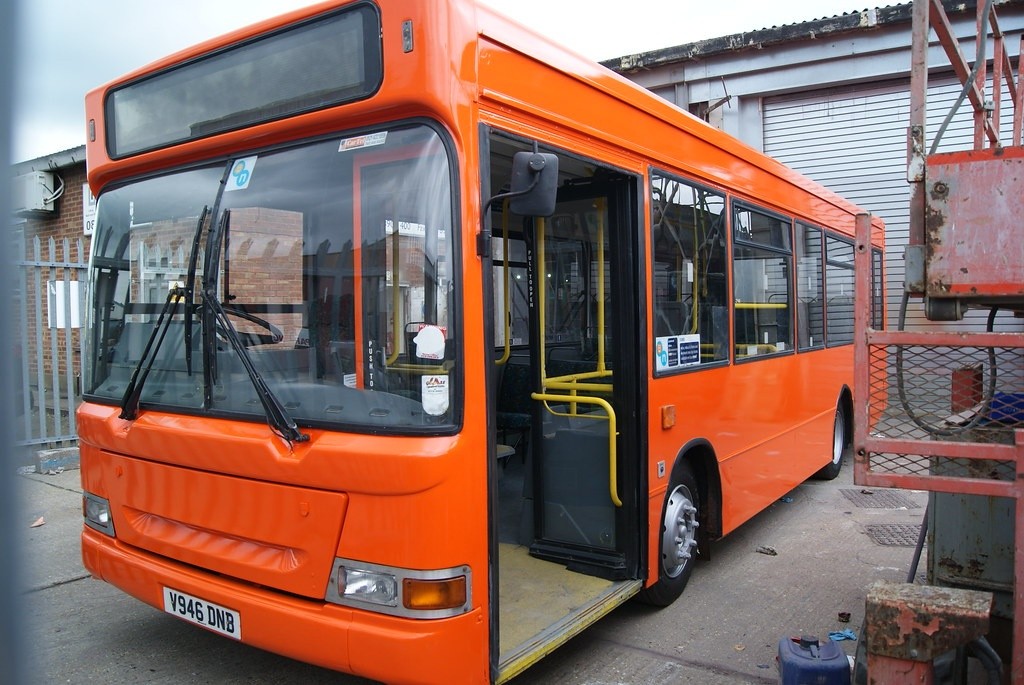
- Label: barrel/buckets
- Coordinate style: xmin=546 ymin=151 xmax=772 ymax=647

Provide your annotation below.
xmin=777 ymin=634 xmax=851 ymax=685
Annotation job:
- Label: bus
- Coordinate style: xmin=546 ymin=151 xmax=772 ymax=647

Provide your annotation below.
xmin=72 ymin=1 xmax=893 ymax=684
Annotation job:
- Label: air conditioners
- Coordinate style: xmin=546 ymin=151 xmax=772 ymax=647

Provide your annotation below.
xmin=9 ymin=171 xmax=54 ymax=217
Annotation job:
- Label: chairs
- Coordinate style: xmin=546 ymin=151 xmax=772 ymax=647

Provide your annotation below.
xmin=404 ymin=293 xmax=854 ymax=470
xmin=293 ymin=293 xmax=355 ymax=379
